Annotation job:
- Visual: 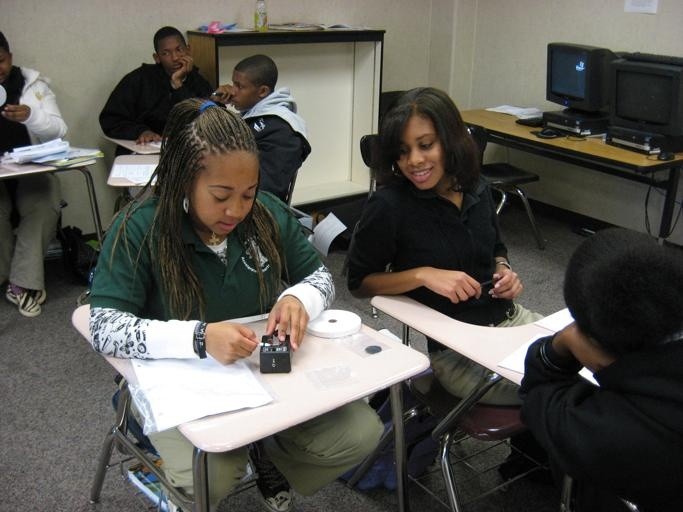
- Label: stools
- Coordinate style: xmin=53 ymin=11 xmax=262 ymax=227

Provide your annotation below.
xmin=477 ymin=158 xmax=547 ymax=254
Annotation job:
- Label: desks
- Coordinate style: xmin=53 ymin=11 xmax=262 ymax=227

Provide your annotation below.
xmin=106 ymin=151 xmax=171 ymax=219
xmin=70 ymin=303 xmax=433 ymax=511
xmin=0 ymin=143 xmax=105 ymax=253
xmin=342 ymin=290 xmax=601 ymax=511
xmin=455 ymin=104 xmax=681 ymax=247
xmin=103 ymin=136 xmax=170 ymax=154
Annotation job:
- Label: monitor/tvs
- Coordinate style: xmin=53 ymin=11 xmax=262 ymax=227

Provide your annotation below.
xmin=611 ymin=53 xmax=683 ymax=138
xmin=546 ymin=42 xmax=616 ymax=117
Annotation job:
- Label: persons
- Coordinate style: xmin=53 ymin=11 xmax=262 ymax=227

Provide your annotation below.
xmin=348 ymin=88 xmax=547 ymax=404
xmin=211 ymin=54 xmax=311 ymax=203
xmin=519 ymin=227 xmax=683 ymax=512
xmin=0 ymin=30 xmax=67 ymax=318
xmin=90 ymin=97 xmax=384 ymax=512
xmin=99 ymin=27 xmax=227 ymax=201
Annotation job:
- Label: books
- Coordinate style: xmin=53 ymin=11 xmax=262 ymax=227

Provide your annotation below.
xmin=44 ymin=152 xmax=104 ymax=167
xmin=268 ymin=24 xmax=321 ymax=31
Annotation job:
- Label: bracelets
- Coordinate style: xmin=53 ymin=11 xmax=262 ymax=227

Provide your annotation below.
xmin=196 ymin=322 xmax=208 ymax=359
xmin=496 ymin=261 xmax=512 ymax=270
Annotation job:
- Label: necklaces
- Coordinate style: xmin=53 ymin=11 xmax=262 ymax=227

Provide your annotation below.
xmin=208 ymin=232 xmax=220 ymax=245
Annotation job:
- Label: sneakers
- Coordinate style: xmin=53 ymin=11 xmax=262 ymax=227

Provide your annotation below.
xmin=247 ymin=441 xmax=295 ymax=512
xmin=5 ymin=283 xmax=42 ymax=318
xmin=28 ymin=288 xmax=47 ymax=305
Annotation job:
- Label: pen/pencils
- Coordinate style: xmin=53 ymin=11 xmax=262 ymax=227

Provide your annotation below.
xmin=256 ymin=343 xmax=280 ymax=347
xmin=480 ymin=276 xmax=503 ymax=288
xmin=212 ymin=92 xmax=224 ymax=96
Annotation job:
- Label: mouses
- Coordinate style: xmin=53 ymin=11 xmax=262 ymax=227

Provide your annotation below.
xmin=542 ymin=128 xmax=559 ymax=136
xmin=658 ymin=151 xmax=675 ymax=160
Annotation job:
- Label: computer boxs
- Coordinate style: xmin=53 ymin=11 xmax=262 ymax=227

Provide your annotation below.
xmin=605 ymin=125 xmax=683 ymax=155
xmin=543 ymin=110 xmax=611 ymax=135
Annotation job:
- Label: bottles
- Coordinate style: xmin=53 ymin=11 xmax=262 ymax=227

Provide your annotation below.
xmin=253 ymin=1 xmax=267 ymax=32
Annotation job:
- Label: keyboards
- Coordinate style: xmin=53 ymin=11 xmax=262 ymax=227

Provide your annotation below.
xmin=625 ymin=53 xmax=683 ymax=67
xmin=516 ymin=117 xmax=543 ymax=128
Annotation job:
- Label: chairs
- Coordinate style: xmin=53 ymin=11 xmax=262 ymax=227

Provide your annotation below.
xmin=346 ymin=124 xmax=540 ymax=508
xmin=82 ymin=270 xmax=260 ymax=512
